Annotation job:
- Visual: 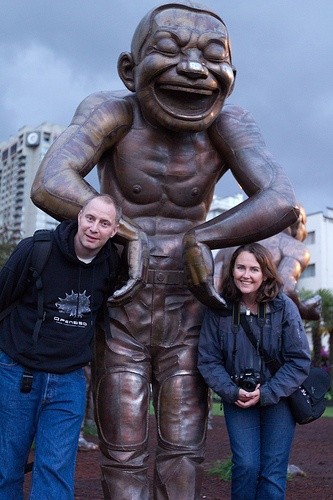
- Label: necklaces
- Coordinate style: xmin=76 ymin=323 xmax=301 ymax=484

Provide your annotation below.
xmin=242 ymin=301 xmax=256 ymax=315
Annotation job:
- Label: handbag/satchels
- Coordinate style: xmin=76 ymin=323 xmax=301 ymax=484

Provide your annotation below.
xmin=271 ymin=364 xmax=329 ymax=425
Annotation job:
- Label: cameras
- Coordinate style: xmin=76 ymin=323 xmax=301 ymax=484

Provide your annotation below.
xmin=231 ymin=368 xmax=265 ymax=392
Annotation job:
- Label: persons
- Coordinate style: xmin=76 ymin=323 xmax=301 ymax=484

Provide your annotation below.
xmin=198 ymin=243 xmax=311 ymax=500
xmin=0 ymin=194 xmax=121 ymax=500
xmin=207 ymin=205 xmax=322 ymax=429
xmin=77 ymin=362 xmax=99 ymax=451
xmin=31 ymin=2 xmax=299 ymax=500
